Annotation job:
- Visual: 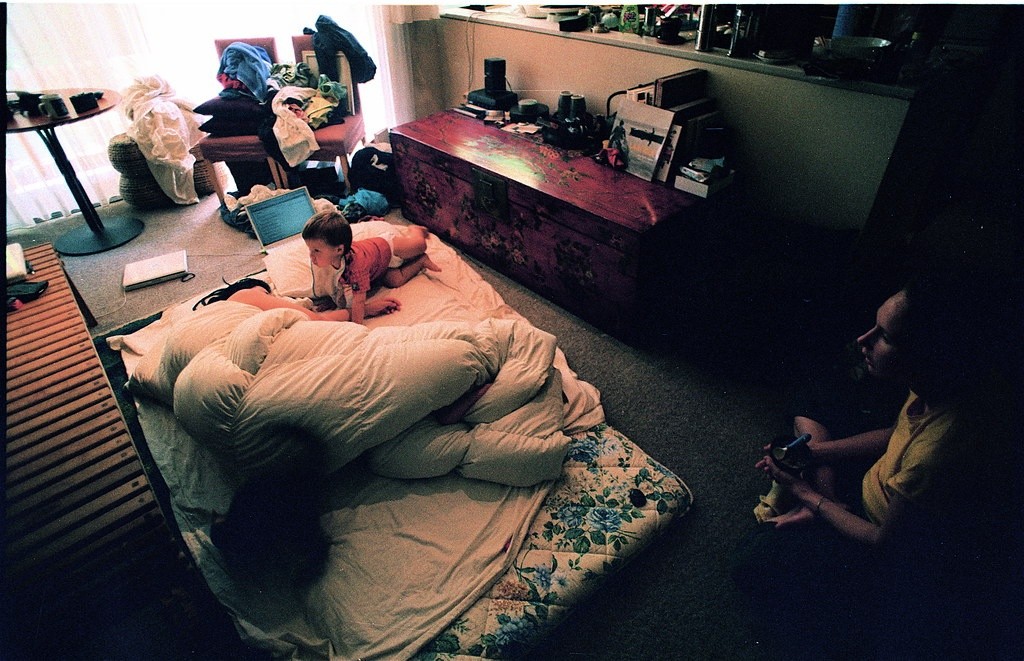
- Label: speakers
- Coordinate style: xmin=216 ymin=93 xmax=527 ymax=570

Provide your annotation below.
xmin=483 ymin=57 xmax=507 ymax=95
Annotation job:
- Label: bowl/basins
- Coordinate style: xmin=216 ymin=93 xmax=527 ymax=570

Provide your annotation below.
xmin=823 ymin=36 xmax=891 ymax=62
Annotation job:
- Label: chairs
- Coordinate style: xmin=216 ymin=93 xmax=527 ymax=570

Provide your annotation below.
xmin=199 ymin=34 xmax=368 ymax=209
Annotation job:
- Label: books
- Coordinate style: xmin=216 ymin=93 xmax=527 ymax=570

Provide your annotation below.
xmin=654 ymin=68 xmax=721 ymax=160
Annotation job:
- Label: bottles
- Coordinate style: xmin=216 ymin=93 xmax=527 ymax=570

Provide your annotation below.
xmin=618 ymin=5 xmax=639 ymax=34
xmin=906 ymin=31 xmax=920 ymax=53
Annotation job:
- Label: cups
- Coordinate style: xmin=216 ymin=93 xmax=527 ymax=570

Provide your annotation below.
xmin=557 ymin=89 xmax=585 ymax=118
xmin=38 ymin=93 xmax=69 ymax=119
xmin=644 ymin=6 xmax=656 ymax=28
xmin=660 ymin=15 xmax=681 ymax=43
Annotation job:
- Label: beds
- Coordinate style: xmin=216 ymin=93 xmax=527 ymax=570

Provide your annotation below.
xmin=90 ymin=210 xmax=694 ymax=661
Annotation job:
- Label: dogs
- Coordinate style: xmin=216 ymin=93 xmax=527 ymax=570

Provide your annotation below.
xmin=212 ymin=420 xmax=332 ymax=596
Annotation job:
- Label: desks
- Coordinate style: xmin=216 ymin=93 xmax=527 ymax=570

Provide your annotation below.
xmin=4 ymin=85 xmax=144 ymax=255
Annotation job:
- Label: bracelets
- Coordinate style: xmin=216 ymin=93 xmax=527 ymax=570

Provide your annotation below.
xmin=814 ymin=495 xmax=829 ymax=515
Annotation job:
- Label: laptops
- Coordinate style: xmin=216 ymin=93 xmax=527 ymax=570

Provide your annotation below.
xmin=244 ymin=185 xmax=317 ymax=255
xmin=123 ymin=249 xmax=189 ymax=292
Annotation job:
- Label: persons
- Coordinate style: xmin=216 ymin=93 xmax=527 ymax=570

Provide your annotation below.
xmin=754 ymin=286 xmax=965 ymax=553
xmin=193 ymin=278 xmax=492 ymax=425
xmin=302 ymin=210 xmax=442 ymax=325
xmin=607 ymin=121 xmax=626 ymax=151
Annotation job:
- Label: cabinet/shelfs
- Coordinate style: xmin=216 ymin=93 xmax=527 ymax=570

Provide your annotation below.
xmin=390 ymin=110 xmax=750 ymax=351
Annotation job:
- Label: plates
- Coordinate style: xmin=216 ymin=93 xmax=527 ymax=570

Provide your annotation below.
xmin=753 ymin=52 xmax=795 ymax=65
